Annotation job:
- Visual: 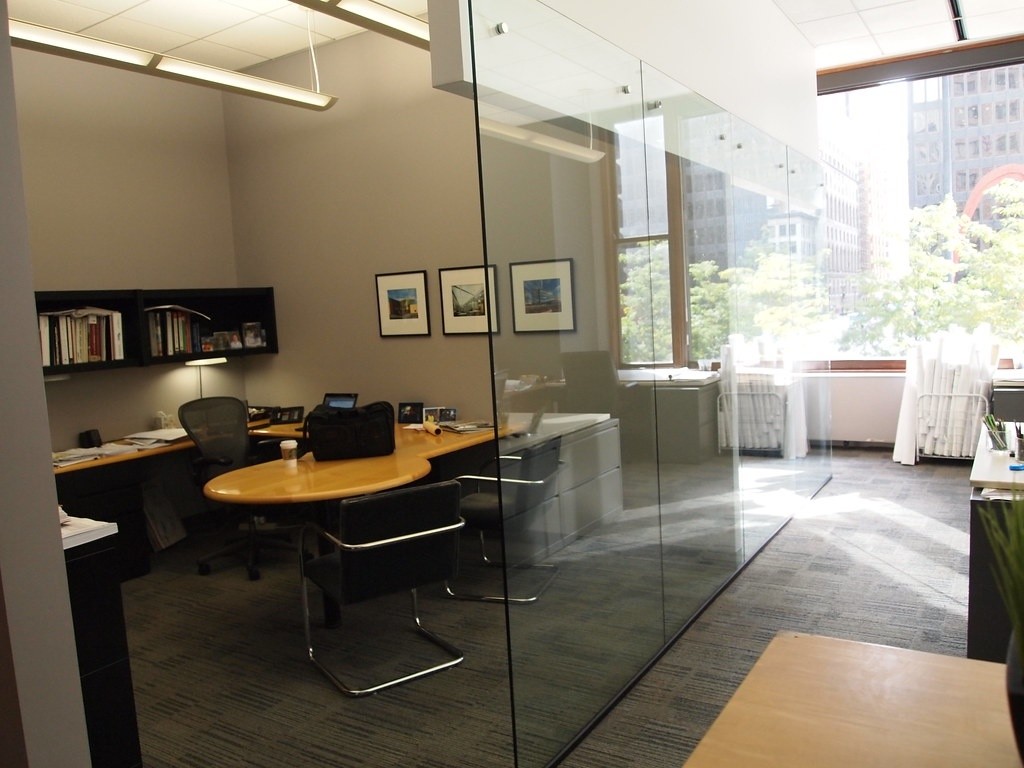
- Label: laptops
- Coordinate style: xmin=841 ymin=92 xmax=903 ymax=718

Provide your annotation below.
xmin=296 ymin=393 xmax=358 ymax=431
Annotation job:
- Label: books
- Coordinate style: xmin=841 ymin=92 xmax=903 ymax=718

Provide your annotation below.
xmin=53 ymin=455 xmax=101 ymax=468
xmin=146 ymin=304 xmax=211 ymax=355
xmin=37 ymin=308 xmax=124 ymax=366
xmin=439 ymin=422 xmax=495 ymax=434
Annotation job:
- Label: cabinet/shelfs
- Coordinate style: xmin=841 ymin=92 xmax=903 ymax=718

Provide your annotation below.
xmin=968 ymin=422 xmax=1024 ymax=665
xmin=34 ymin=287 xmax=279 ymax=377
xmin=616 ymin=388 xmax=721 ymax=466
xmin=468 ymin=412 xmax=624 ymax=571
xmin=992 ymin=387 xmax=1024 ymax=422
xmin=58 ymin=515 xmax=145 ymax=768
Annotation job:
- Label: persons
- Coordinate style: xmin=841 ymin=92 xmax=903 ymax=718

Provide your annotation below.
xmin=202 ymin=336 xmax=214 ymax=352
xmin=230 ymin=333 xmax=242 ymax=349
xmin=214 ymin=333 xmax=228 ymax=350
xmin=443 ymin=410 xmax=450 ymax=421
xmin=425 ymin=410 xmax=435 ymax=423
xmin=244 ymin=326 xmax=263 ymax=347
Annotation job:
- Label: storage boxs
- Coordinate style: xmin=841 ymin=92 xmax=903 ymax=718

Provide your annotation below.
xmin=140 ymin=463 xmax=187 ymax=555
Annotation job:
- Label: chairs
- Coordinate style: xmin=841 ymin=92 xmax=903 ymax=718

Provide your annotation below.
xmin=560 ymin=351 xmax=651 ymax=465
xmin=297 ymin=478 xmax=467 ymax=699
xmin=437 ymin=435 xmax=567 ymax=605
xmin=178 ymin=396 xmax=312 ymax=583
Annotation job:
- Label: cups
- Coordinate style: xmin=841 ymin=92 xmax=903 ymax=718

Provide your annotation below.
xmin=280 ymin=440 xmax=298 ymax=468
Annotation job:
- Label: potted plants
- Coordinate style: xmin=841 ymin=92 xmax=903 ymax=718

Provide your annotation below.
xmin=976 ymin=470 xmax=1024 ymax=768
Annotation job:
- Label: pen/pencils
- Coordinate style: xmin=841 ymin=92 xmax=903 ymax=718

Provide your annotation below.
xmin=982 ymin=414 xmax=1007 ymax=450
xmin=1014 ymin=419 xmax=1024 ymax=460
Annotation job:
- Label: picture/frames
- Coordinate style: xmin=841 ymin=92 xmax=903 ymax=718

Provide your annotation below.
xmin=440 ymin=409 xmax=456 ymax=421
xmin=374 ymin=270 xmax=431 ymax=338
xmin=201 ymin=336 xmax=226 ymax=352
xmin=272 ymin=406 xmax=303 ymax=423
xmin=243 ymin=322 xmax=263 ymax=349
xmin=399 ymin=403 xmax=424 ymax=423
xmin=423 ymin=407 xmax=445 ymax=424
xmin=509 ymin=257 xmax=577 ymax=334
xmin=438 ymin=265 xmax=501 ymax=334
xmin=210 ymin=330 xmax=242 ymax=350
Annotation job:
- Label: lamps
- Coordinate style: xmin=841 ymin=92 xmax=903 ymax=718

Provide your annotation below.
xmin=479 ymin=107 xmax=604 ymax=165
xmin=8 ymin=17 xmax=339 ymax=113
xmin=286 ymin=0 xmax=430 ymax=52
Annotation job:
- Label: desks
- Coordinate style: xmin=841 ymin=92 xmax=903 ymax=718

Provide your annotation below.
xmin=247 ymin=420 xmax=513 ymax=461
xmin=682 ymin=626 xmax=1024 ymax=768
xmin=54 ymin=416 xmax=274 ymax=583
